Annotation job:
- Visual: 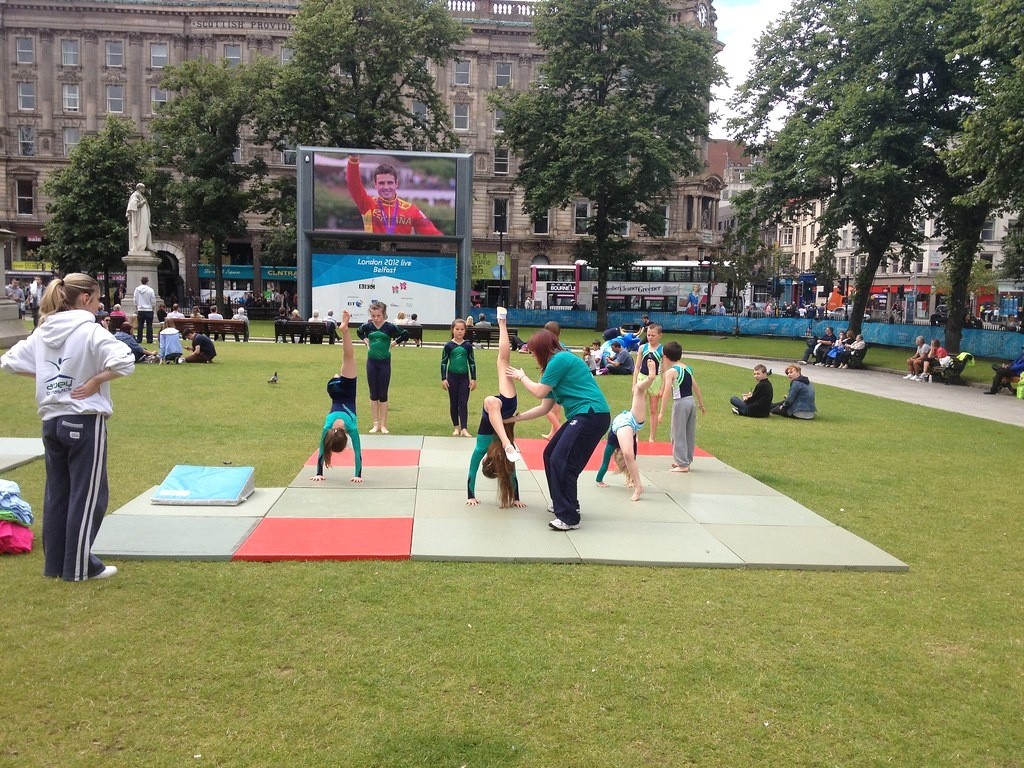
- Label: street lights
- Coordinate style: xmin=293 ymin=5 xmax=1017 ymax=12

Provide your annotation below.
xmin=697 ymin=242 xmax=727 ymax=314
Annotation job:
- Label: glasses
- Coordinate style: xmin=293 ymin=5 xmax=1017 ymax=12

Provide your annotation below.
xmin=106 ymin=319 xmax=111 ymax=321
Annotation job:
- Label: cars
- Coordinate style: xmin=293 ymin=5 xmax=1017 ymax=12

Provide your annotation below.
xmin=797 ymin=305 xmax=826 ymax=320
xmin=827 ymin=306 xmax=871 ymax=322
xmin=770 ymin=305 xmax=791 ymax=318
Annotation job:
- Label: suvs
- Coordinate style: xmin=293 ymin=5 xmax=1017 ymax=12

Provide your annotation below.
xmin=930 ymin=304 xmax=984 ymax=330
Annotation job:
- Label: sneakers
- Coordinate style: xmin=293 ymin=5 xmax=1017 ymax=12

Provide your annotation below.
xmin=548 ymin=519 xmax=581 ymax=531
xmin=548 ymin=500 xmax=580 ymax=513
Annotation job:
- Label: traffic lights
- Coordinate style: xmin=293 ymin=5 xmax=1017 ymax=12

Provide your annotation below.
xmin=806 ymin=282 xmax=812 ymax=293
xmin=837 ymin=278 xmax=845 ymax=296
xmin=897 ymin=284 xmax=905 ymax=298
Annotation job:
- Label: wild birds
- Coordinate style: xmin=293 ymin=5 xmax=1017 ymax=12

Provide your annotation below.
xmin=267 ymin=372 xmax=278 ymax=384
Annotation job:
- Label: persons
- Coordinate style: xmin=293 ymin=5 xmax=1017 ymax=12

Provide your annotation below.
xmin=475 ymin=313 xmax=491 ymax=328
xmin=5 ymin=276 xmax=305 ymax=365
xmin=465 ymin=315 xmax=474 ymax=327
xmin=581 ymin=315 xmax=654 ymax=376
xmin=595 ymin=359 xmax=656 ymax=501
xmin=687 ymin=284 xmax=699 ymax=313
xmin=126 ymin=183 xmax=156 ymax=251
xmin=541 ymin=321 xmax=561 ymax=441
xmin=465 ymin=307 xmax=528 ymax=508
xmin=708 ymin=300 xmax=1024 ymax=399
xmin=658 ymin=342 xmax=705 ymax=472
xmin=394 ymin=311 xmax=423 ymax=347
xmin=632 ymin=324 xmax=669 ymax=442
xmin=346 ymin=153 xmax=444 ymax=237
xmin=275 ymin=308 xmax=343 ymax=344
xmin=730 ymin=364 xmax=774 ymax=418
xmin=0 ymin=273 xmax=135 ymax=581
xmin=356 ymin=301 xmax=409 ymax=433
xmin=310 ymin=309 xmax=364 ymax=483
xmin=770 ymin=365 xmax=817 ymax=420
xmin=505 ymin=329 xmax=611 ymax=531
xmin=440 ymin=318 xmax=476 ymax=437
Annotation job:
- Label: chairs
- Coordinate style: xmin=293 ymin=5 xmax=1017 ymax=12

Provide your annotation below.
xmin=591 ymin=273 xmax=597 ymax=279
xmin=649 ymin=273 xmax=655 ymax=281
xmin=673 ymin=273 xmax=677 ymax=281
xmin=661 ymin=274 xmax=664 ymax=280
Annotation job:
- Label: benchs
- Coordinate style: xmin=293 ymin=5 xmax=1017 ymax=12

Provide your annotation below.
xmin=811 ymin=343 xmax=872 ymax=370
xmin=274 ymin=319 xmax=336 ymax=344
xmin=913 ymin=352 xmax=973 ymax=385
xmin=452 ymin=327 xmax=519 ymax=349
xmin=164 ymin=317 xmax=250 ymax=343
xmin=997 ymin=359 xmax=1023 ymax=394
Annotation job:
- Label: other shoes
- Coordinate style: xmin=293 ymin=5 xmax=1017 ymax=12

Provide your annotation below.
xmin=91 ymin=566 xmax=118 ymax=578
xmin=732 ymin=407 xmax=739 ymax=415
xmin=903 ymin=373 xmax=929 ymax=383
xmin=497 ymin=306 xmax=507 ymax=319
xmin=799 ymin=361 xmax=848 ymax=369
xmin=992 ymin=365 xmax=1002 ymax=371
xmin=505 ymin=445 xmax=520 ymax=462
xmin=175 ymin=357 xmax=179 ymax=364
xmin=984 ymin=391 xmax=999 ymax=394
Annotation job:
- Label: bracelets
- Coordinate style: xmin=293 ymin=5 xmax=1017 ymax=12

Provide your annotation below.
xmin=520 ymin=375 xmax=526 ymax=381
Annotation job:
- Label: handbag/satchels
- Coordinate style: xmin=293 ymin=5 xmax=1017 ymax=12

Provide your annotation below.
xmin=1017 ymin=371 xmax=1024 ymax=399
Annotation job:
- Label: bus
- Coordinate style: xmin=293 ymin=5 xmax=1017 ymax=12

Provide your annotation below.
xmin=573 ymin=259 xmax=755 ymax=317
xmin=529 ymin=264 xmax=665 ymax=311
